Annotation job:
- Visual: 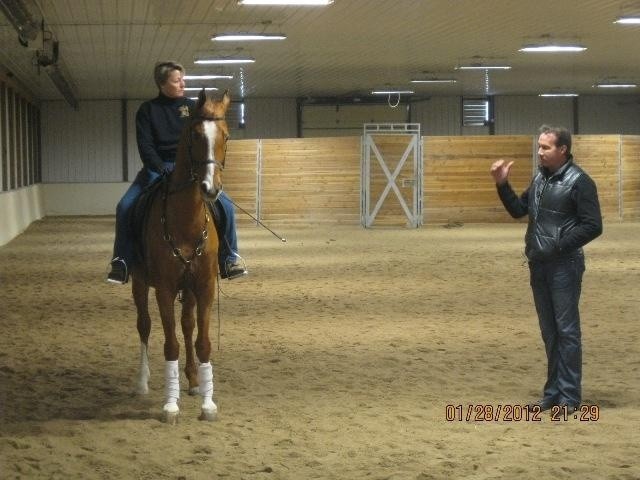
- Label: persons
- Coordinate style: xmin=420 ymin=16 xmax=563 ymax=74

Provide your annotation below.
xmin=105 ymin=59 xmax=246 ymax=280
xmin=490 ymin=123 xmax=602 ymax=416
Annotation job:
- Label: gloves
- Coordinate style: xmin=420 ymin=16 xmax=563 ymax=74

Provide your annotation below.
xmin=156 ymin=165 xmax=171 ymax=176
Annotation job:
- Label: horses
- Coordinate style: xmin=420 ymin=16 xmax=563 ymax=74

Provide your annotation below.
xmin=129 ymin=87 xmax=230 ymax=425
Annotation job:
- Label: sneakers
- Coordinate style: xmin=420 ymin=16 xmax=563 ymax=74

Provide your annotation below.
xmin=108 ymin=263 xmax=129 ymax=283
xmin=219 ymin=261 xmax=245 ymax=278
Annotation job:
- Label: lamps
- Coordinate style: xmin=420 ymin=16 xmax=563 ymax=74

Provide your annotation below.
xmin=371 ymin=16 xmax=640 ymax=96
xmin=194 ymin=0 xmax=336 ymax=66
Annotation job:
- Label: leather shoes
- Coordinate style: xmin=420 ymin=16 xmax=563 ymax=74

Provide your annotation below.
xmin=527 ymin=399 xmax=576 ymax=416
